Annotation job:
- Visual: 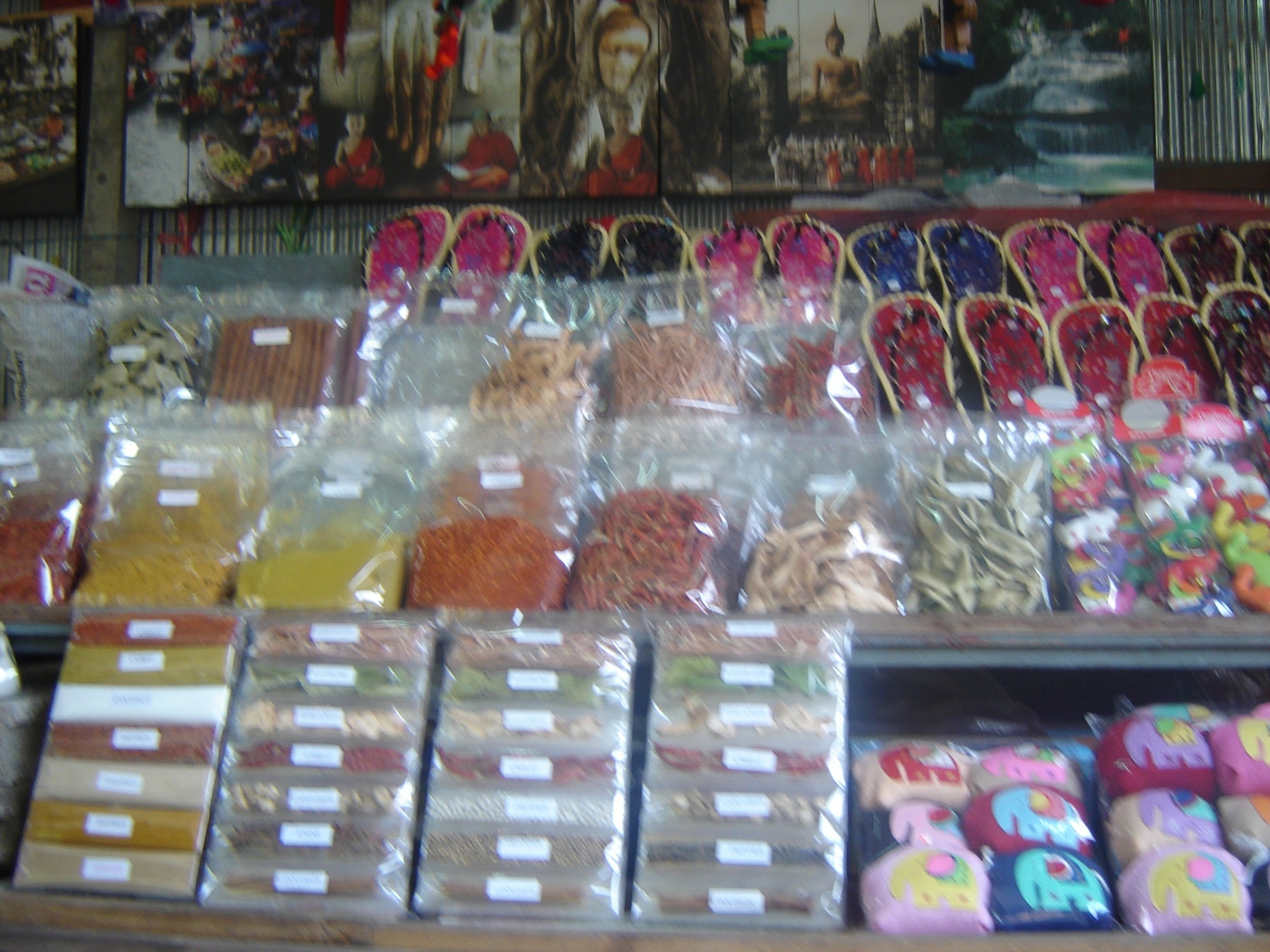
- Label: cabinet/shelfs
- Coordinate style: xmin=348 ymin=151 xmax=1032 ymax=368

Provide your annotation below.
xmin=0 ymin=603 xmax=1270 ymax=952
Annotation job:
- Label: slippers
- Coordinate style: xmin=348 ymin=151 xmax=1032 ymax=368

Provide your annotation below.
xmin=609 ymin=214 xmax=689 ymax=324
xmin=438 ymin=202 xmax=531 ymax=328
xmin=532 ymin=218 xmax=609 ymax=330
xmin=360 ymin=204 xmax=453 ymax=323
xmin=689 ymin=211 xmax=1270 ymax=433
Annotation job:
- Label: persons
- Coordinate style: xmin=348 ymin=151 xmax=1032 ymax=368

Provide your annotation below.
xmin=0 ymin=0 xmax=979 ymax=197
xmin=803 ymin=10 xmax=872 ymax=108
